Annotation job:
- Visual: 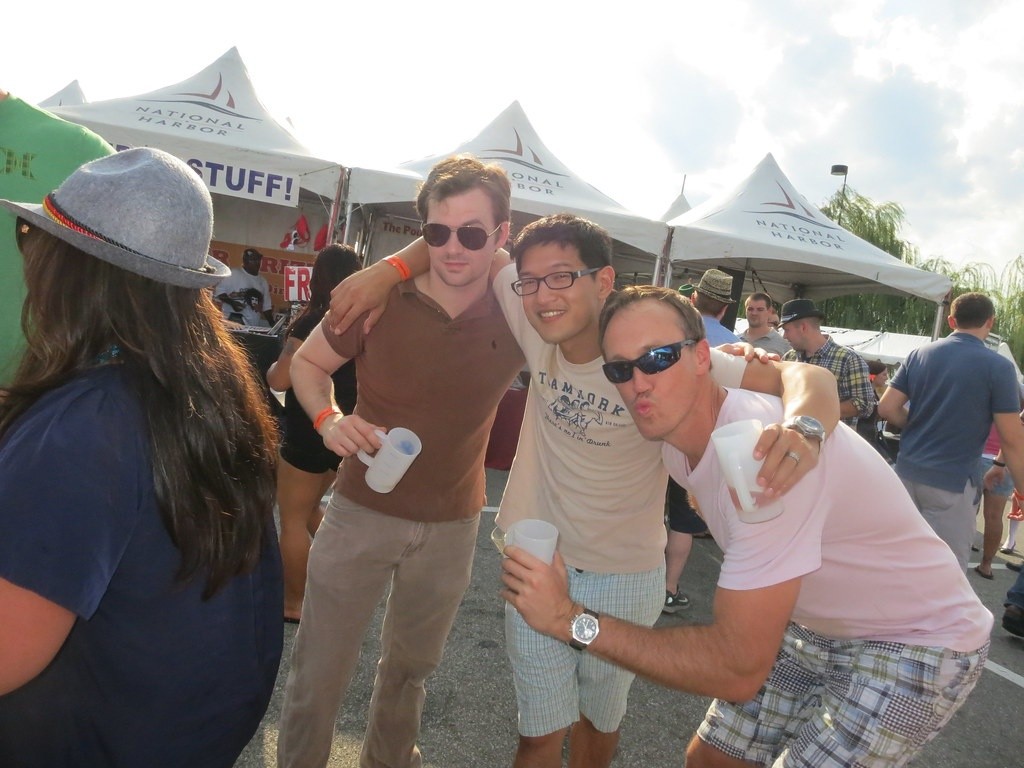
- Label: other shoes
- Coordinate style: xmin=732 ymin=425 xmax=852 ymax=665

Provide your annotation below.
xmin=283 ymin=617 xmax=300 ymax=624
xmin=1006 ymin=561 xmax=1022 ymax=572
xmin=1000 ymin=541 xmax=1015 ymax=554
xmin=1002 ymin=605 xmax=1024 ymax=638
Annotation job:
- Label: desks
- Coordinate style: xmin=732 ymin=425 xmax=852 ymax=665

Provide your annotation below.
xmin=228 ymin=328 xmax=284 ymax=389
xmin=485 ymin=389 xmax=528 ymax=472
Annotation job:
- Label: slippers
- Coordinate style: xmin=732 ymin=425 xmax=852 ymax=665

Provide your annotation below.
xmin=975 ymin=565 xmax=994 ymax=578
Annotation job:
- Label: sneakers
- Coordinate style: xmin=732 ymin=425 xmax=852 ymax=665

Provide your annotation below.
xmin=663 ymin=584 xmax=694 ymax=613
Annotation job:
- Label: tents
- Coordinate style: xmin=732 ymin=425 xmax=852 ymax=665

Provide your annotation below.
xmin=731 ymin=318 xmax=1024 ymax=395
xmin=31 ymin=45 xmax=954 ymax=313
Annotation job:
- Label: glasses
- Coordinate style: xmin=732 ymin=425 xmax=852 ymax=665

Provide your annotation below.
xmin=767 ymin=321 xmax=778 ymax=326
xmin=15 ymin=216 xmax=33 ymax=254
xmin=420 ymin=218 xmax=502 ymax=250
xmin=602 ymin=338 xmax=698 ymax=383
xmin=511 ymin=265 xmax=606 ymax=296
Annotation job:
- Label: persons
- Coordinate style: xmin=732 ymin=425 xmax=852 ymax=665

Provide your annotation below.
xmin=265 ymin=246 xmax=376 ymax=625
xmin=878 ymin=292 xmax=1024 ymax=581
xmin=0 ymin=146 xmax=284 ymax=768
xmin=277 ymin=158 xmax=780 ymax=768
xmin=1002 ymin=565 xmax=1024 ymax=638
xmin=213 ymin=248 xmax=277 ymax=332
xmin=0 ymin=88 xmax=122 ymax=426
xmin=329 ymin=216 xmax=840 ymax=768
xmin=610 ymin=270 xmax=1024 ymax=613
xmin=502 ymin=285 xmax=995 ymax=768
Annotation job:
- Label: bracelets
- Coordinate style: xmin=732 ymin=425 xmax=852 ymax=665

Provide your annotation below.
xmin=1013 ymin=488 xmax=1024 ymax=500
xmin=382 ymin=255 xmax=411 ymax=281
xmin=314 ymin=406 xmax=342 ymax=432
xmin=992 ymin=460 xmax=1005 ymax=467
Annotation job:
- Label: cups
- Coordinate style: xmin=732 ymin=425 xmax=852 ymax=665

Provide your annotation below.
xmin=504 ymin=518 xmax=558 ymax=565
xmin=711 ymin=419 xmax=782 ymax=523
xmin=357 ymin=427 xmax=422 ymax=493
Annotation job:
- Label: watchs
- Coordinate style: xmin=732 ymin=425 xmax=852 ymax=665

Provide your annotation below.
xmin=569 ymin=608 xmax=600 ymax=652
xmin=780 ymin=415 xmax=825 ymax=454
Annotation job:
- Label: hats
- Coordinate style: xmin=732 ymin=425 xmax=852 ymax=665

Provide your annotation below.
xmin=0 ymin=146 xmax=232 ymax=290
xmin=692 ymin=268 xmax=736 ymax=303
xmin=776 ymin=299 xmax=824 ymax=329
xmin=678 ymin=284 xmax=694 ymax=296
xmin=243 ymin=248 xmax=262 ymax=260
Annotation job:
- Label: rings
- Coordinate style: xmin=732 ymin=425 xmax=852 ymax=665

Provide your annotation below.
xmin=785 ymin=451 xmax=800 ymax=465
